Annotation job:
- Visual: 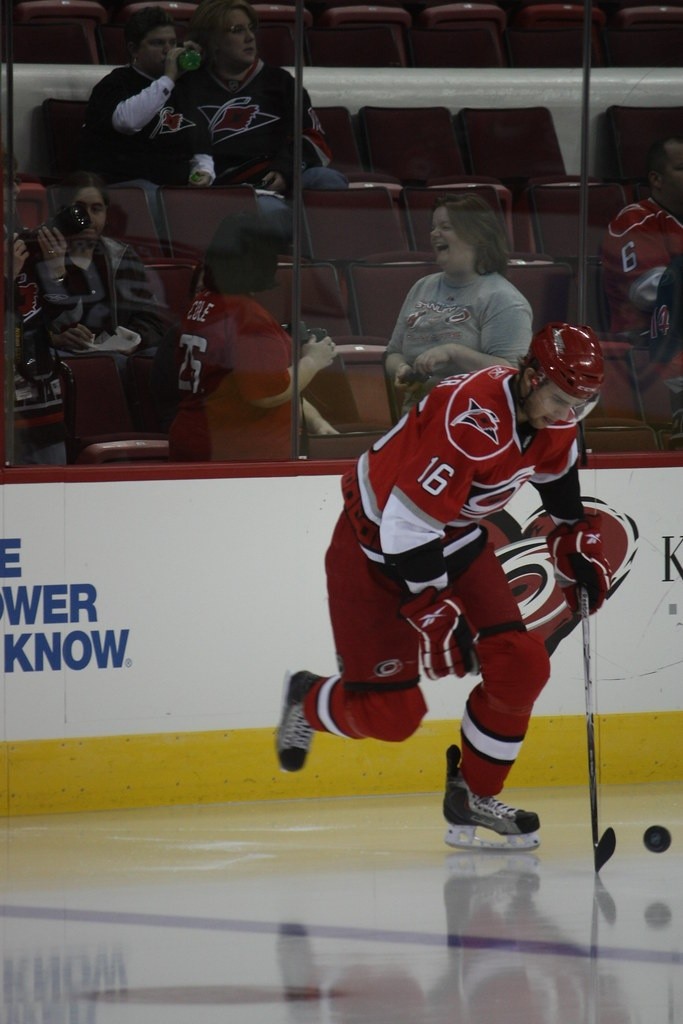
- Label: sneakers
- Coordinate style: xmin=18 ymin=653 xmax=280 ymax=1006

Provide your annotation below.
xmin=442 ymin=745 xmax=539 ymax=849
xmin=275 ymin=670 xmax=326 ymax=772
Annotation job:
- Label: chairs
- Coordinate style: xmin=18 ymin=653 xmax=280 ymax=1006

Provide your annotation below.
xmin=0 ymin=0 xmax=683 ymax=459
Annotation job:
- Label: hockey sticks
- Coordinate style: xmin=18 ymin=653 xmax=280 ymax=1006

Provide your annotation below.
xmin=580 ymin=584 xmax=616 ymax=872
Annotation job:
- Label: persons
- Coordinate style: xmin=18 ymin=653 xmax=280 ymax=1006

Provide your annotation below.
xmin=276 ymin=851 xmax=630 ymax=1023
xmin=277 ymin=319 xmax=640 ymax=848
xmin=597 ymin=135 xmax=683 ymax=452
xmin=0 ymin=146 xmax=170 ymax=465
xmin=178 ymin=217 xmax=340 ymax=460
xmin=74 ymin=1 xmax=351 ymax=189
xmin=378 ymin=192 xmax=534 ymax=423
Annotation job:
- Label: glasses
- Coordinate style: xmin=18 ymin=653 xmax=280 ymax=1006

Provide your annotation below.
xmin=224 ymin=25 xmax=256 ymax=34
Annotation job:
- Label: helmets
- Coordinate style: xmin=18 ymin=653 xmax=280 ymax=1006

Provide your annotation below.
xmin=530 ymin=321 xmax=603 ymax=400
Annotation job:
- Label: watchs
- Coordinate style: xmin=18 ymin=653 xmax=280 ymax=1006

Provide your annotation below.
xmin=49 ymin=274 xmax=65 ymax=287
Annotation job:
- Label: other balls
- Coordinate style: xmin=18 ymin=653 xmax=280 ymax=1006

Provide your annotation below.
xmin=642 ymin=824 xmax=673 ymax=853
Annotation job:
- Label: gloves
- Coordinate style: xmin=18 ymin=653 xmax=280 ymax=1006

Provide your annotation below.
xmin=398 ymin=586 xmax=475 ymax=681
xmin=547 ymin=518 xmax=611 ymax=616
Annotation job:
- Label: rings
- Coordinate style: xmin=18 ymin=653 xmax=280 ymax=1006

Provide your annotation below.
xmin=328 ymin=343 xmax=334 ymax=351
xmin=48 ymin=250 xmax=54 ymax=254
